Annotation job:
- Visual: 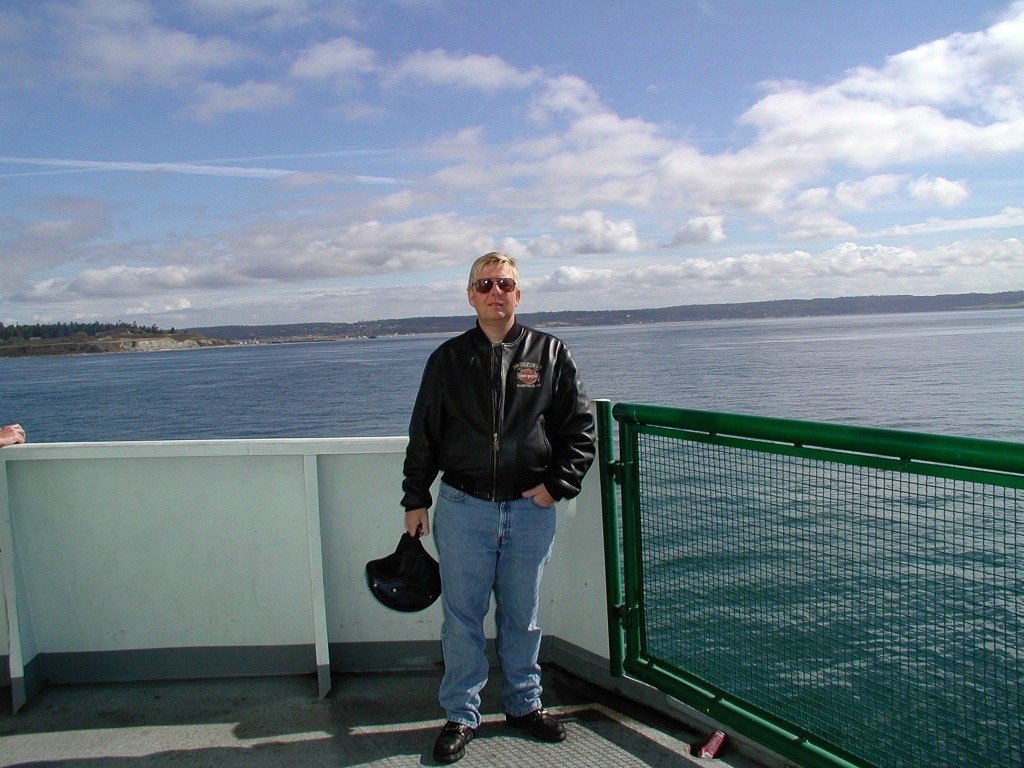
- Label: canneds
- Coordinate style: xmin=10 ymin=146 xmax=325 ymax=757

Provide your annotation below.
xmin=697 ymin=730 xmax=726 ymax=760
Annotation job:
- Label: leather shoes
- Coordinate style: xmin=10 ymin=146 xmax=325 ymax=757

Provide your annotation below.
xmin=435 ymin=720 xmax=475 ymax=763
xmin=504 ymin=709 xmax=566 ymax=743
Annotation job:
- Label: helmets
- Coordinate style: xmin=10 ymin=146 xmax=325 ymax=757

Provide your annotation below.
xmin=366 ymin=522 xmax=442 ymax=612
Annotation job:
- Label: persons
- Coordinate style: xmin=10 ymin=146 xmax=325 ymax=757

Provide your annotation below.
xmin=0 ymin=423 xmax=26 ymax=448
xmin=369 ymin=252 xmax=598 ymax=765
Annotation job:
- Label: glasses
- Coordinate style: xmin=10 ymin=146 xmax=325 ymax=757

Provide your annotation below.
xmin=471 ymin=278 xmax=516 ymax=293
